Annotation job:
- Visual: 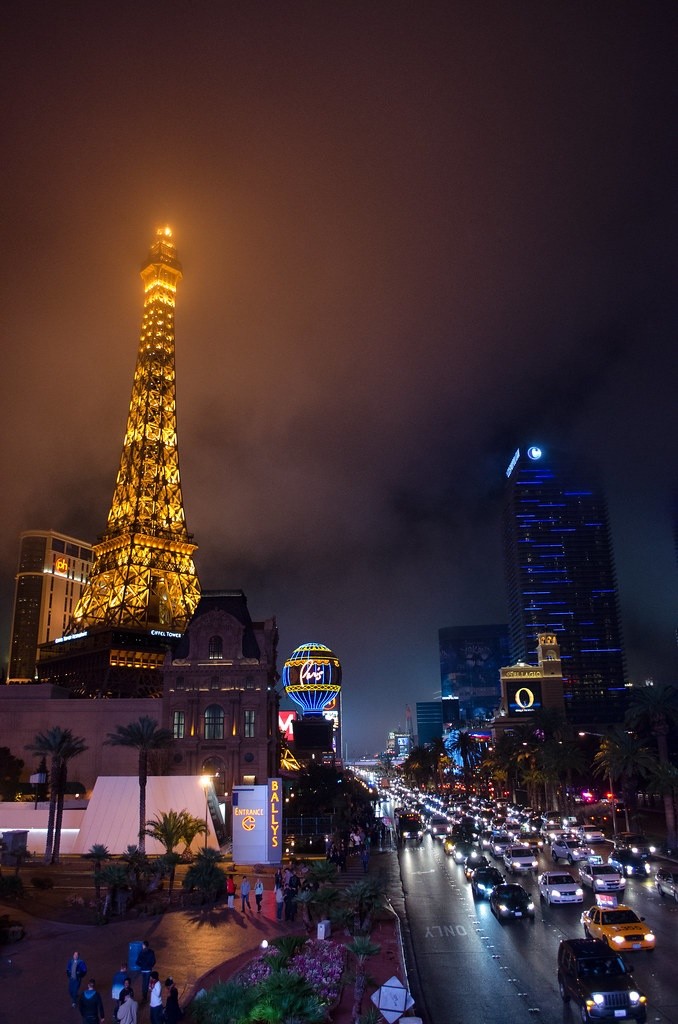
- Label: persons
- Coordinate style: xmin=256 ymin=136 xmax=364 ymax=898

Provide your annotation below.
xmin=163 ymin=986 xmax=183 ymax=1024
xmin=111 ymin=961 xmax=130 ymax=1024
xmin=79 ymin=978 xmax=105 ymax=1024
xmin=325 ymin=824 xmax=366 ymax=872
xmin=240 ymin=876 xmax=252 ymax=912
xmin=65 ymin=952 xmax=87 ymax=1009
xmin=116 ymin=994 xmax=138 ymax=1024
xmin=119 ymin=977 xmax=134 ymax=1008
xmin=274 ymin=868 xmax=302 ymax=922
xmin=225 ymin=875 xmax=236 ymax=912
xmin=149 ymin=971 xmax=163 ymax=1024
xmin=128 ymin=938 xmax=143 ymax=975
xmin=135 ymin=940 xmax=157 ymax=1004
xmin=254 ymin=878 xmax=263 ymax=913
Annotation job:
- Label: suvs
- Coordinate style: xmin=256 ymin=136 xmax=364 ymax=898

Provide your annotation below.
xmin=556 ymin=937 xmax=648 ymax=1024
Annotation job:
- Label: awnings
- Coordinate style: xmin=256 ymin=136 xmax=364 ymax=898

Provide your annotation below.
xmin=276 ymin=768 xmax=304 ymax=779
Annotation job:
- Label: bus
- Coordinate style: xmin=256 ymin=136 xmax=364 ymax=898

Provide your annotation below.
xmin=395 ymin=810 xmax=424 ymax=844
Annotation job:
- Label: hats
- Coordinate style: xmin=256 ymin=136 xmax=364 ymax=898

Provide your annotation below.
xmin=165 ymin=976 xmax=173 ymax=986
xmin=151 ymin=971 xmax=159 ymax=980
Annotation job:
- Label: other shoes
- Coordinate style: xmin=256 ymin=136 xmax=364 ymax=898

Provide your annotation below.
xmin=72 ymin=1003 xmax=77 ymax=1008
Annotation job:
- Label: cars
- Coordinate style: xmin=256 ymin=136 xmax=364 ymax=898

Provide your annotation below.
xmin=613 ymin=832 xmax=654 ymax=858
xmin=574 ymin=793 xmax=631 ymax=815
xmin=490 ymin=883 xmax=535 ymax=925
xmin=488 ymin=834 xmax=516 ymax=860
xmin=655 ymin=866 xmax=678 ymax=903
xmin=374 ymin=776 xmax=582 ymax=855
xmin=472 ymin=866 xmax=507 ymax=900
xmin=551 ymin=839 xmax=595 ymax=866
xmin=464 ymin=855 xmax=492 ymax=881
xmin=579 ymin=863 xmax=626 ymax=895
xmin=503 ymin=846 xmax=539 ymax=876
xmin=582 ymin=904 xmax=656 ymax=954
xmin=452 ymin=842 xmax=478 ymax=865
xmin=608 ymin=849 xmax=651 ymax=878
xmin=538 ymin=871 xmax=584 ymax=907
xmin=576 ymin=825 xmax=605 ymax=844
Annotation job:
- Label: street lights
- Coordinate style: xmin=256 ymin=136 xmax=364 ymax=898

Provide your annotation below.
xmin=578 ymin=732 xmax=617 ymax=834
xmin=203 ymin=777 xmax=210 ymax=849
